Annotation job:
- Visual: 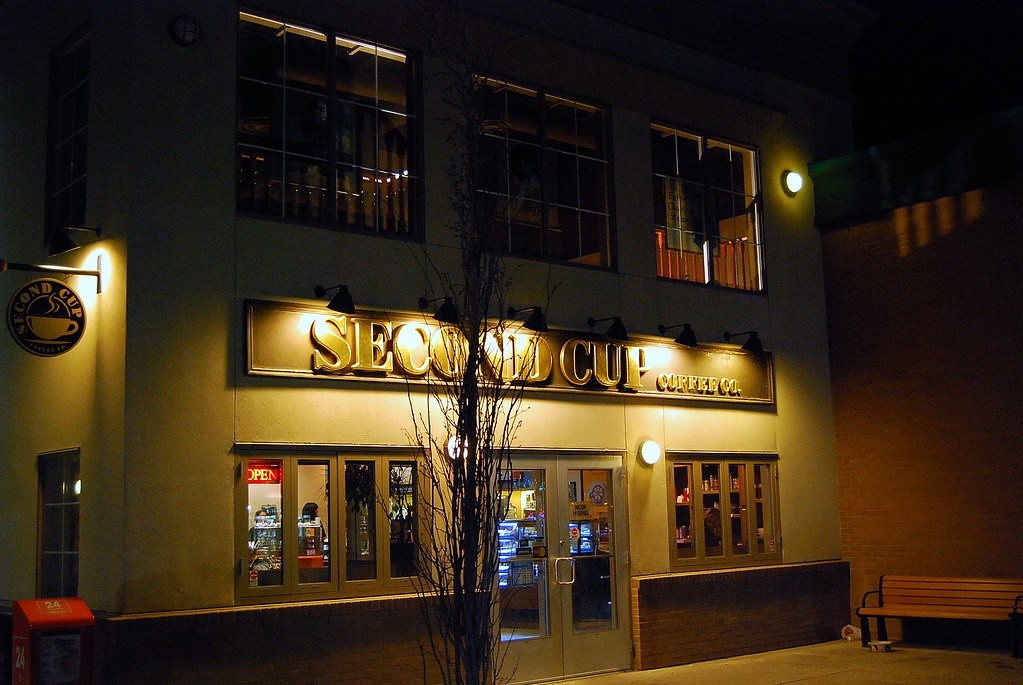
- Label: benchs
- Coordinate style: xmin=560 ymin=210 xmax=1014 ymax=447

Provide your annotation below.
xmin=856 ymin=573 xmax=1023 ymax=655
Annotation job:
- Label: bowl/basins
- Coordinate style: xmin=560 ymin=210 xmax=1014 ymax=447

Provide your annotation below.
xmin=868 ymin=641 xmax=892 ymax=653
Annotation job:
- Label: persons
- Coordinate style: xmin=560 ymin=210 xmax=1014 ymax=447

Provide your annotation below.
xmin=248 ymin=510 xmax=267 ymax=547
xmin=302 ymin=502 xmax=326 ymax=540
xmin=704 ymin=508 xmax=722 ymax=548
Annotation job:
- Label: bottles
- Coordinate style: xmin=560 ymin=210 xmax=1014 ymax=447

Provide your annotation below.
xmin=703 ymin=474 xmax=719 ymax=490
xmin=730 ymin=474 xmax=740 ymax=489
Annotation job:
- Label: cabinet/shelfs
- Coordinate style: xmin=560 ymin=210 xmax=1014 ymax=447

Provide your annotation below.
xmin=253 ymin=517 xmax=323 ymax=558
xmin=665 ymin=450 xmax=783 ymax=572
xmin=499 ymin=517 xmax=600 ymax=613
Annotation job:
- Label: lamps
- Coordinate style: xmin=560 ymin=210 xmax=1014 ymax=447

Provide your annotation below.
xmin=658 ymin=323 xmax=697 ymax=347
xmin=781 ymin=169 xmax=802 ymax=198
xmin=46 ymin=219 xmax=103 ymax=256
xmin=587 ymin=315 xmax=629 ymax=340
xmin=507 ymin=305 xmax=549 ymax=332
xmin=443 ymin=435 xmax=468 ymax=461
xmin=638 ymin=439 xmax=660 ymax=466
xmin=724 ymin=331 xmax=763 ymax=354
xmin=314 ymin=284 xmax=355 ymax=314
xmin=417 ymin=296 xmax=460 ymax=322
